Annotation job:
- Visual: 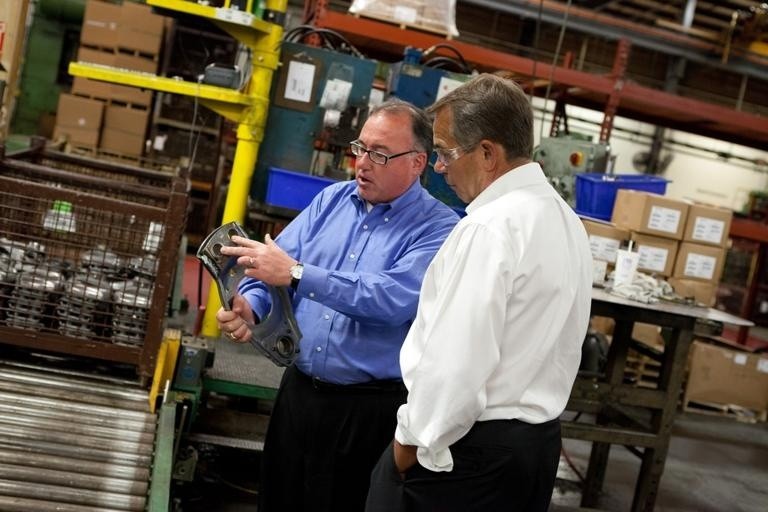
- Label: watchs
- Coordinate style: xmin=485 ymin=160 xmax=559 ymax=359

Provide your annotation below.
xmin=289 ymin=260 xmax=302 ymax=289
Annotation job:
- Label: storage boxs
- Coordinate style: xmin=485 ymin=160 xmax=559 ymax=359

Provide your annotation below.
xmin=265 ymin=166 xmax=337 ymax=211
xmin=573 ymin=174 xmax=733 ymax=308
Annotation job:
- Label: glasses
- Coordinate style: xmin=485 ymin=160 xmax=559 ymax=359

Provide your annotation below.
xmin=349 ymin=139 xmax=418 ymax=166
xmin=432 ymin=143 xmax=473 ymax=167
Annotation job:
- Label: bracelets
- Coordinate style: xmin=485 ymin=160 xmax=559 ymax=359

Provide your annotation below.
xmin=230 ymin=332 xmax=237 ymax=340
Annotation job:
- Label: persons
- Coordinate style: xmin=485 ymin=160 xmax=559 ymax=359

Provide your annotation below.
xmin=217 ymin=98 xmax=461 ymax=512
xmin=362 ymin=70 xmax=595 ymax=512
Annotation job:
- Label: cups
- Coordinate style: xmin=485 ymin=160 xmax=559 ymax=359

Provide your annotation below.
xmin=614 ymin=249 xmax=640 ymax=287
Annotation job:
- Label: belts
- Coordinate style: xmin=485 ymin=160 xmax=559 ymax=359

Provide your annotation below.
xmin=288 ymin=364 xmax=402 ymax=396
